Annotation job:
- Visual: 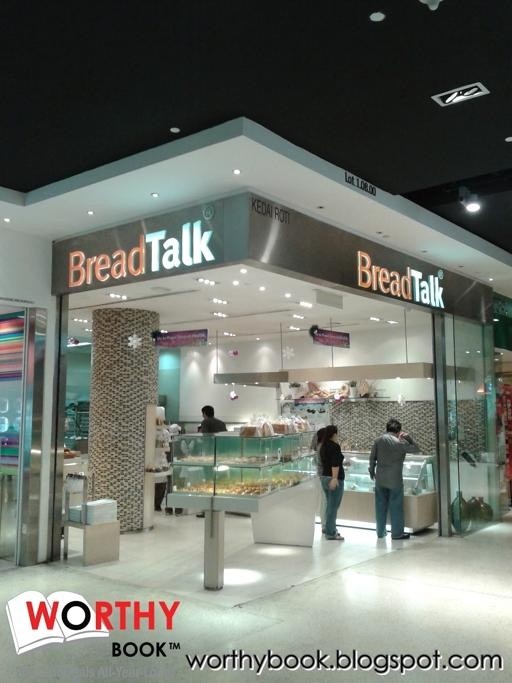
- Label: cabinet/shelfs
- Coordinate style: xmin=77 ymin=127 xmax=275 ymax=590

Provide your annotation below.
xmin=165 ymin=428 xmax=300 ymax=515
xmin=317 ymin=458 xmax=436 ymax=530
xmin=68 ymin=411 xmax=91 ymax=452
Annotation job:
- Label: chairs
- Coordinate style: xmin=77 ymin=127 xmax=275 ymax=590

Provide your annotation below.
xmin=63 ymin=472 xmax=121 ymax=565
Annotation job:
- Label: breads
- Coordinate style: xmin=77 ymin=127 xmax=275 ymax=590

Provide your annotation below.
xmin=239 ymin=420 xmax=312 ymax=439
xmin=180 ymin=478 xmax=300 ymax=495
xmin=181 ymin=453 xmax=292 ymax=463
xmin=63 ymin=448 xmax=77 ymax=458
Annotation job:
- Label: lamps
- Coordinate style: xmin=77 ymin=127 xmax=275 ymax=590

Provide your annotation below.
xmin=461 ymin=193 xmax=482 ymax=214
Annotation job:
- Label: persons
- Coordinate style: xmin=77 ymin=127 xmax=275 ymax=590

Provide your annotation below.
xmin=318 ymin=425 xmax=345 ymax=540
xmin=197 ymin=403 xmax=226 ymax=432
xmin=311 ymin=427 xmax=328 ymax=534
xmin=367 ymin=417 xmax=422 ymax=539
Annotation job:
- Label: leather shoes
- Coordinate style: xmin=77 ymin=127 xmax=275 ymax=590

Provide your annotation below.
xmin=391 ymin=532 xmax=410 ymax=539
xmin=321 ymin=529 xmax=344 ymax=541
